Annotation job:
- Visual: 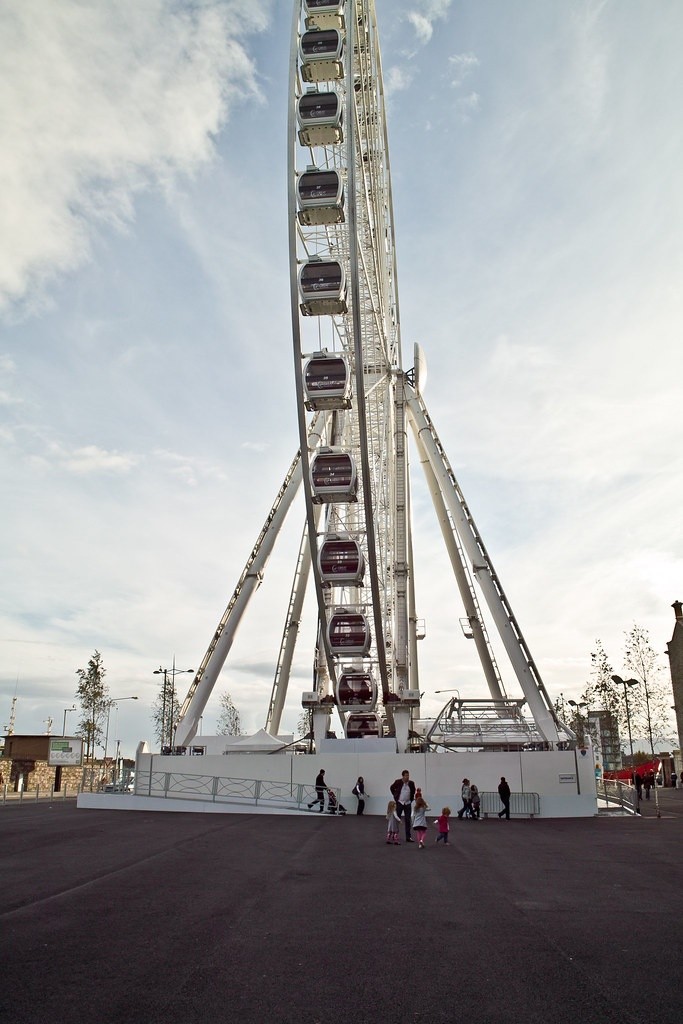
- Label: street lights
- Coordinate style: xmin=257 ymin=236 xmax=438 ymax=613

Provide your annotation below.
xmin=611 ymin=673 xmax=641 ymax=815
xmin=569 ymin=699 xmax=587 ymax=715
xmin=200 ymin=716 xmax=203 ymax=736
xmin=105 ymin=695 xmax=138 ymax=756
xmin=114 ymin=740 xmax=122 ymax=771
xmin=435 ymin=688 xmax=460 ymax=699
xmin=153 ymin=668 xmax=168 ymax=755
xmin=170 ymin=667 xmax=195 ymax=753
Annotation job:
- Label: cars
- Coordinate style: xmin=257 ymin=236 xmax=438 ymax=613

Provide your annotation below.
xmin=106 ymin=776 xmax=135 ymax=794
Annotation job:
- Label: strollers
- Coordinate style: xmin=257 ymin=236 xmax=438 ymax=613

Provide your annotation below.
xmin=325 ymin=789 xmax=348 ymax=816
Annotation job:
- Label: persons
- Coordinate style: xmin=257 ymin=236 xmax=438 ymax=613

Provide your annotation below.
xmin=411 ymin=797 xmax=431 ymax=849
xmin=670 ymin=771 xmax=677 ymax=789
xmin=680 ymin=772 xmax=683 ymax=783
xmin=457 ymin=778 xmax=484 ymax=821
xmin=631 ymin=768 xmax=662 ymax=801
xmin=339 ymin=682 xmax=351 ymax=705
xmin=498 ymin=777 xmax=511 ymax=820
xmin=358 ymin=719 xmax=370 ymax=739
xmin=307 ymin=769 xmax=330 ymax=814
xmin=390 ymin=770 xmax=415 ymax=842
xmin=385 ymin=801 xmax=403 ymax=849
xmin=356 ymin=681 xmax=369 ymax=705
xmin=354 ymin=777 xmax=369 ymax=816
xmin=415 ymin=788 xmax=422 ymax=800
xmin=433 ymin=808 xmax=451 ymax=846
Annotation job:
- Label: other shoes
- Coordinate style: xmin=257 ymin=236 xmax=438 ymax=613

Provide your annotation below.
xmin=319 ymin=810 xmax=325 ymax=813
xmin=445 ymin=843 xmax=450 ymax=846
xmin=306 ymin=805 xmax=311 ymax=810
xmin=418 ymin=840 xmax=424 ymax=848
xmin=435 ymin=836 xmax=437 ymax=842
xmin=394 ymin=842 xmax=401 ymax=845
xmin=406 ymin=837 xmax=415 ymax=842
xmin=386 ymin=841 xmax=393 ymax=844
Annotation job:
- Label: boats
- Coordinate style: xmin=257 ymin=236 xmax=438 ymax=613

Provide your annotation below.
xmin=594 ymin=759 xmax=664 ymax=787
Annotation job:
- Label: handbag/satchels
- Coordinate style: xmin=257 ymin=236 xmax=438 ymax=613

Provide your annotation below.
xmin=352 ymin=783 xmax=359 ymax=795
